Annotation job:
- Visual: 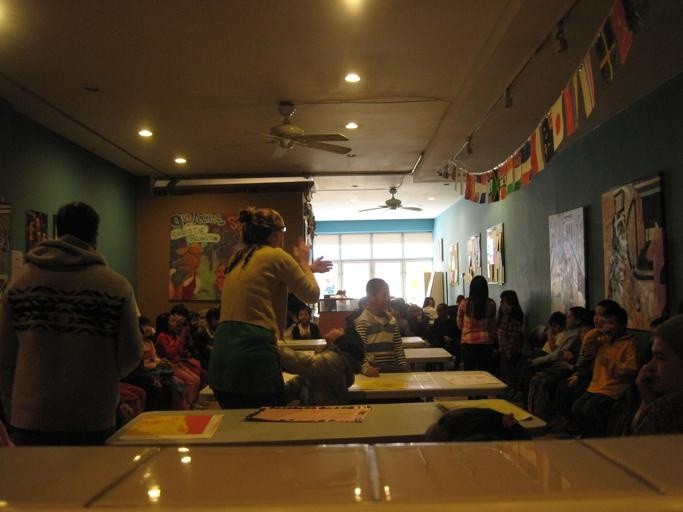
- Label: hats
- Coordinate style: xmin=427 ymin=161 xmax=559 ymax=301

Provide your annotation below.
xmin=655 ymin=315 xmax=683 ymax=361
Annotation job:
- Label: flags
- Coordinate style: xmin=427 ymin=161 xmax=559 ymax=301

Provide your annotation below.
xmin=464 ymin=0 xmax=635 ymax=203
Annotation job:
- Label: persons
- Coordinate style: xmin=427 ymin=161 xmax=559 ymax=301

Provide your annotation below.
xmin=345 ymin=297 xmax=368 ymax=334
xmin=389 ymin=275 xmax=683 ymax=443
xmin=292 ymin=306 xmax=321 ymax=340
xmin=208 ymin=206 xmax=333 ymax=408
xmin=0 ymin=202 xmax=143 ymax=446
xmin=272 ymin=327 xmax=364 ymax=407
xmin=354 ymin=279 xmax=410 ymax=377
xmin=117 ymin=303 xmax=221 ymax=426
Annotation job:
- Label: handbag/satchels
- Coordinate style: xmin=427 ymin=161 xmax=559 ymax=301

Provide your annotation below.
xmin=426 ymin=408 xmax=533 ymax=442
xmin=150 ymin=364 xmax=174 ymax=377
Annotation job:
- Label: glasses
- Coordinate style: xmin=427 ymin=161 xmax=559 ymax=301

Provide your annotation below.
xmin=275 ymin=226 xmax=287 ymax=232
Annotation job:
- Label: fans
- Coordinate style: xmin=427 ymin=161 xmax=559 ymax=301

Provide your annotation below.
xmin=358 ymin=187 xmax=423 ymax=213
xmin=214 ymin=101 xmax=352 ymax=155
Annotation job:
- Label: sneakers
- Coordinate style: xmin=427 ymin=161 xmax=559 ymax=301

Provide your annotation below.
xmin=190 ymin=399 xmax=209 ymax=409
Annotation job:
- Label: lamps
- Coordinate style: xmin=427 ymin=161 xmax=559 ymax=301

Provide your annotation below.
xmin=503 ymin=87 xmax=512 ymax=108
xmin=464 ymin=136 xmax=472 ymax=157
xmin=552 ymin=19 xmax=568 ymax=52
